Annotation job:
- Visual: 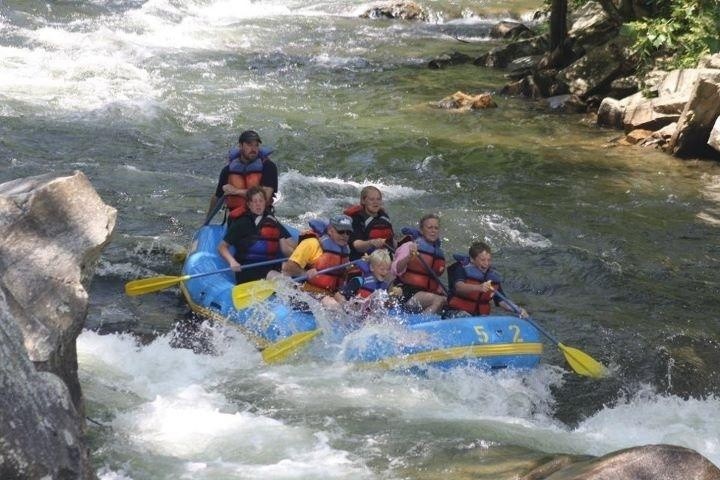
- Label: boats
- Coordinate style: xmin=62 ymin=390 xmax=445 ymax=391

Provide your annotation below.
xmin=180 ymin=222 xmax=545 ymax=377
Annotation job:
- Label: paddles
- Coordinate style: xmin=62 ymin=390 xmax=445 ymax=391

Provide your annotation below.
xmin=487 ymin=280 xmax=608 ymax=377
xmin=260 ymin=284 xmax=401 ymax=364
xmin=232 ymin=256 xmax=370 ymax=310
xmin=126 ymin=258 xmax=287 ymax=296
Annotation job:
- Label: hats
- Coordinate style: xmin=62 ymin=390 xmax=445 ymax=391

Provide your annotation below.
xmin=331 ymin=214 xmax=353 ymax=232
xmin=238 ymin=130 xmax=262 ymax=145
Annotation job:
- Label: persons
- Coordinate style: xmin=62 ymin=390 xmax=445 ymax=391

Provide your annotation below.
xmin=344 ymin=186 xmax=399 ymax=261
xmin=390 ymin=215 xmax=448 ymax=316
xmin=283 ymin=215 xmax=354 ymax=312
xmin=208 ymin=130 xmax=297 ymax=249
xmin=440 ymin=242 xmax=528 ymax=319
xmin=343 ymin=249 xmax=400 ymax=318
xmin=217 ymin=186 xmax=294 ymax=287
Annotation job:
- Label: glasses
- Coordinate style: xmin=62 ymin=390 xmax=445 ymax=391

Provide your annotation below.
xmin=338 ymin=230 xmax=351 ymax=235
